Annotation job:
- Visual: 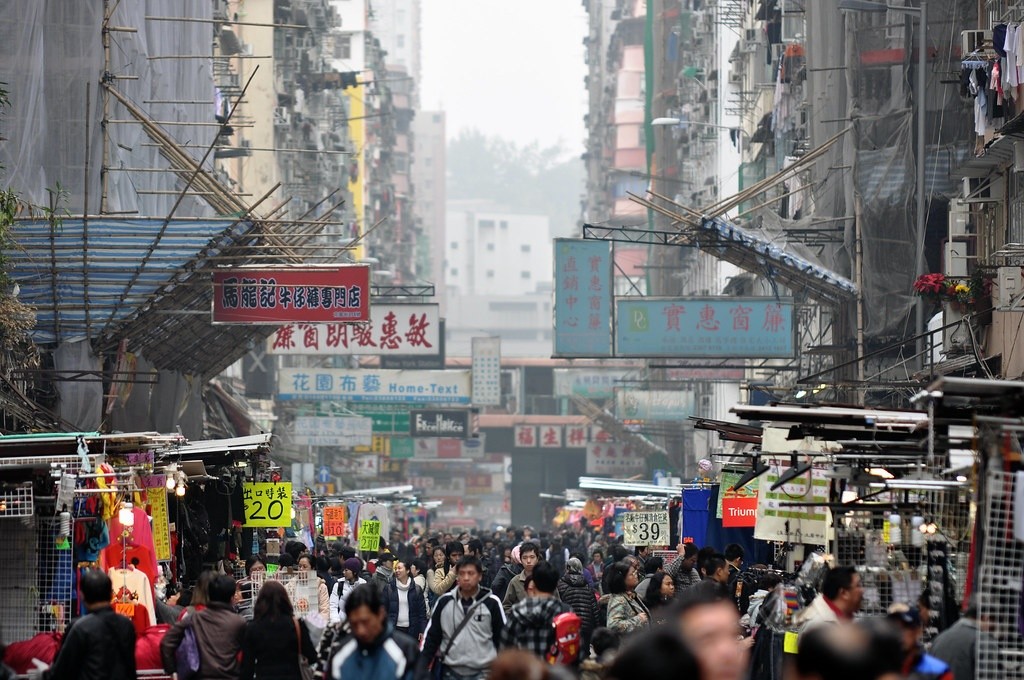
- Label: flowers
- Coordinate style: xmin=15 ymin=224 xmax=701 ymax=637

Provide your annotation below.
xmin=913 ymin=272 xmax=975 ymax=305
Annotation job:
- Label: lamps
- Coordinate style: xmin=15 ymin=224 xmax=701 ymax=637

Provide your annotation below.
xmin=771 ymin=451 xmax=812 ymax=492
xmin=733 ymin=449 xmax=769 ymax=491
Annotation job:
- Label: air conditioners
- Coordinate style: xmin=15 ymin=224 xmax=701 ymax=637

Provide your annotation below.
xmin=944 ymin=30 xmax=1024 ymax=311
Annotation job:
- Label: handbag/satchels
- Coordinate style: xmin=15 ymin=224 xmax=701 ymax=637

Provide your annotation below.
xmin=174 ymin=626 xmax=200 ymax=679
xmin=429 ymin=658 xmax=442 ymax=680
xmin=293 ymin=615 xmax=315 ymax=680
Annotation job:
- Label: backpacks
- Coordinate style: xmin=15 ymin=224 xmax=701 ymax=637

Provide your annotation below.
xmin=546 ymin=612 xmax=582 ymax=667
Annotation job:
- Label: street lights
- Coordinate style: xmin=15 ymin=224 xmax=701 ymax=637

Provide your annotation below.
xmin=649 ymin=116 xmax=753 ymax=165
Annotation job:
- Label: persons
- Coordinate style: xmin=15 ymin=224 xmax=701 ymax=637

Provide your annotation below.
xmin=0 ymin=642 xmax=16 ymax=680
xmin=792 ymin=566 xmax=863 ymax=642
xmin=664 ymin=584 xmax=747 ymax=680
xmin=155 ymin=517 xmax=1024 ymax=680
xmin=386 ymin=561 xmax=427 ymax=650
xmin=234 ymin=581 xmax=319 ymax=680
xmin=27 ymin=568 xmax=139 ymax=680
xmin=328 ymin=557 xmax=367 ymax=631
xmin=419 ymin=554 xmax=509 ymax=680
xmin=323 ymin=583 xmax=417 ymax=680
xmin=160 ymin=576 xmax=248 ymax=680
xmin=692 ymin=459 xmax=714 ymax=490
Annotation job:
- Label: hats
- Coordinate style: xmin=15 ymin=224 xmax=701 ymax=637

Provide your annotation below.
xmin=379 ymin=553 xmax=399 ymax=562
xmin=886 ymin=606 xmax=921 ymax=628
xmin=344 ymin=558 xmax=361 ymax=574
